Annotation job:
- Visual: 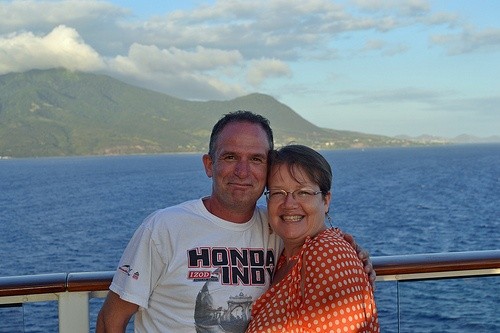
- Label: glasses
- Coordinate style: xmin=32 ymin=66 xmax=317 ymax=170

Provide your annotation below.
xmin=264 ymin=188 xmax=323 ymax=202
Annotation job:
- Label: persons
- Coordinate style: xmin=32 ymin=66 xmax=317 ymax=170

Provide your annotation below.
xmin=245 ymin=144 xmax=382 ymax=333
xmin=96 ymin=110 xmax=378 ymax=333
